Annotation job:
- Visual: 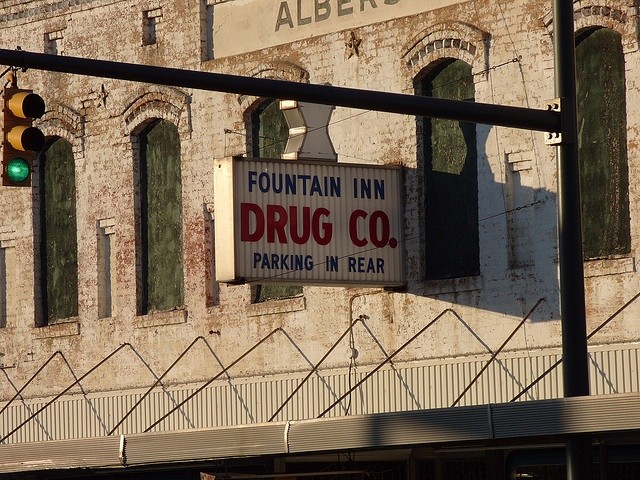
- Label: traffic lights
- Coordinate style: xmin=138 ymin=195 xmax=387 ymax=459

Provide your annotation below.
xmin=2 ymin=87 xmax=46 ymax=187
